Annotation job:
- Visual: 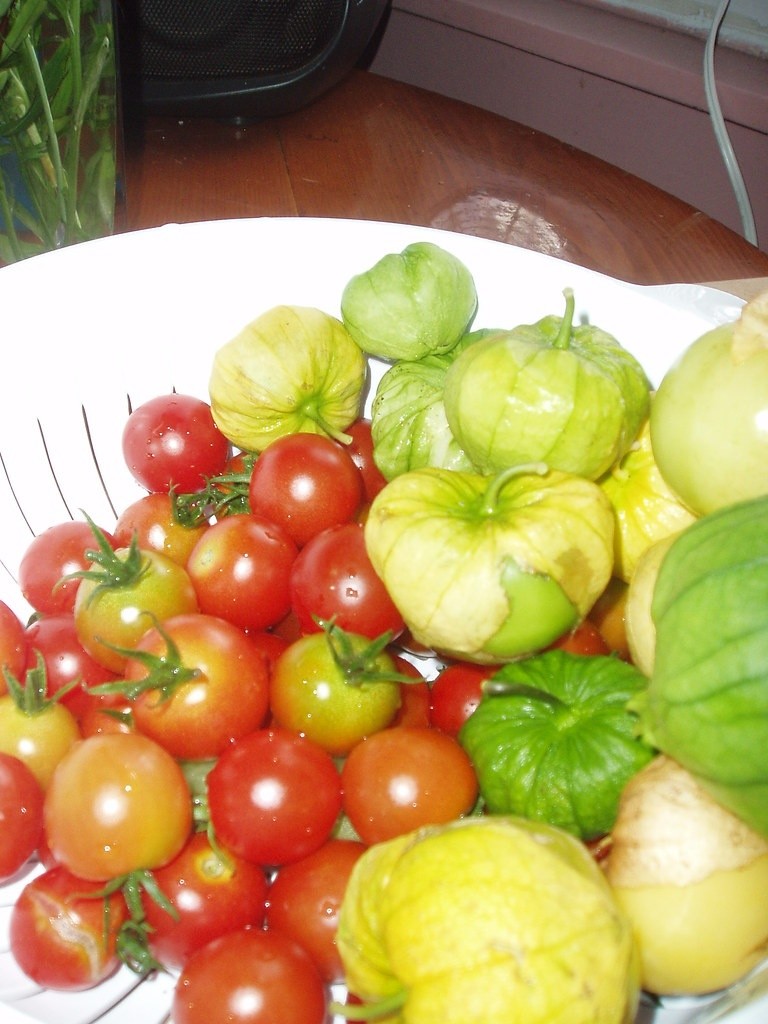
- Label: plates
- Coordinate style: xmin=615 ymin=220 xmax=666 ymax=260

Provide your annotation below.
xmin=0 ymin=216 xmax=767 ymax=1023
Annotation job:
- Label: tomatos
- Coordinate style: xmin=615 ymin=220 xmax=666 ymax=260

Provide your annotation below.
xmin=0 ymin=387 xmax=632 ymax=1024
